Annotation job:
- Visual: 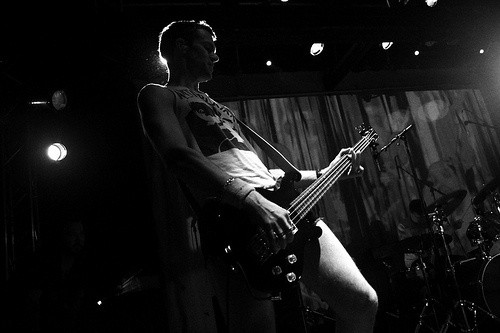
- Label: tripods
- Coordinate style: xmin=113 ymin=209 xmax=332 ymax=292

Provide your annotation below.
xmin=438 ymin=222 xmax=499 ymax=333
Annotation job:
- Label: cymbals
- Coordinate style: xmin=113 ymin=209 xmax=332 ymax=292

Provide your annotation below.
xmin=393 ymin=232 xmax=452 ymax=252
xmin=471 ymin=177 xmax=500 ymax=205
xmin=425 ymin=190 xmax=468 ymax=218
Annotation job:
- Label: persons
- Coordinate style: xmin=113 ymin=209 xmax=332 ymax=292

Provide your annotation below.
xmin=396 ymin=199 xmax=431 ymax=241
xmin=136 ymin=19 xmax=378 ymax=333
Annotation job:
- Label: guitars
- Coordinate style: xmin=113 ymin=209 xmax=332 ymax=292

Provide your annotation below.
xmin=200 ymin=124 xmax=380 ymax=296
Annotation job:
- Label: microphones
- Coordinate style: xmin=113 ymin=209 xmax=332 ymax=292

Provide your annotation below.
xmin=460 ymin=110 xmax=471 ymax=137
xmin=373 ymin=149 xmax=381 ymax=172
xmin=395 ymin=157 xmax=401 ymax=179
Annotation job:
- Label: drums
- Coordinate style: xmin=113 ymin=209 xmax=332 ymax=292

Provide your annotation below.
xmin=465 ymin=219 xmax=495 ymax=246
xmin=421 ymin=245 xmax=469 ymax=281
xmin=474 ymin=253 xmax=500 ymax=321
xmin=479 ymin=190 xmax=500 ymax=216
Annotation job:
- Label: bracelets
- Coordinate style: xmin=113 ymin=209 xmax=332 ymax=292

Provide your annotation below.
xmin=221 ymin=175 xmax=255 ymax=211
xmin=315 ymin=168 xmax=322 ymax=179
